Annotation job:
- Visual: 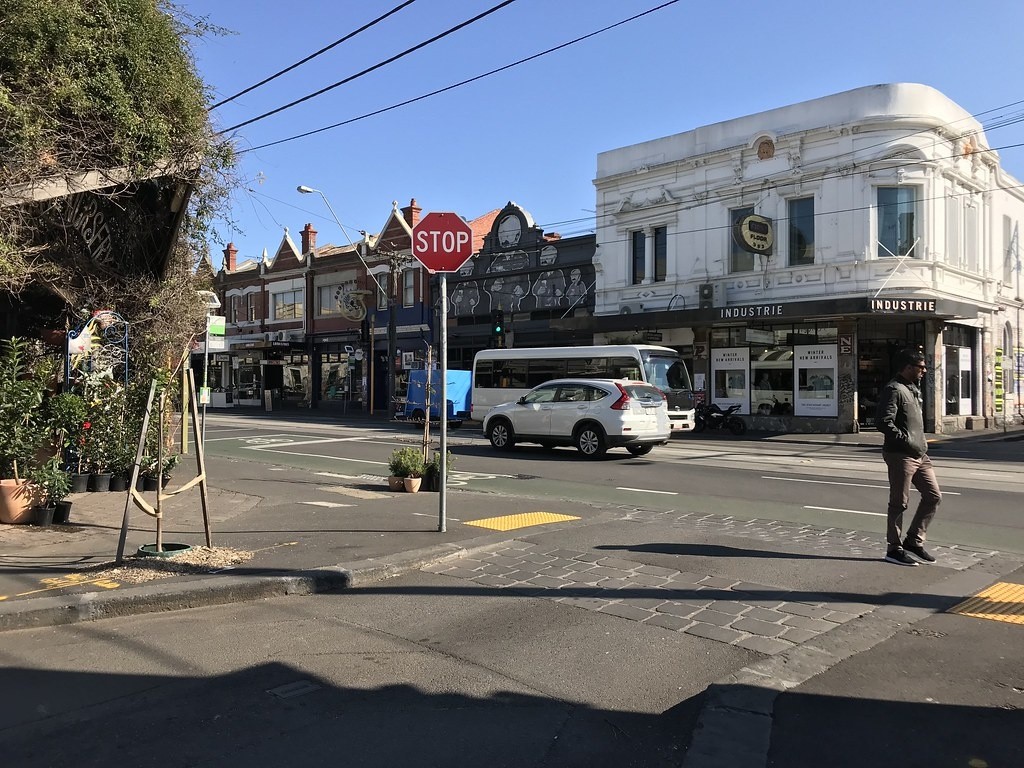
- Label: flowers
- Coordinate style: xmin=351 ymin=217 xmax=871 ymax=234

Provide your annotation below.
xmin=72 ymin=422 xmax=91 ymax=474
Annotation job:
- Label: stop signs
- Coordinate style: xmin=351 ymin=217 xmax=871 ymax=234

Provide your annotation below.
xmin=412 ymin=211 xmax=474 ymax=273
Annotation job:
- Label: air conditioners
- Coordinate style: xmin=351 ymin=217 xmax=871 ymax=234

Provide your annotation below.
xmin=618 ymin=303 xmax=645 ymax=314
xmin=278 ymin=330 xmax=290 ymax=341
xmin=265 ymin=332 xmax=277 ymax=341
xmin=698 ymin=282 xmax=727 ymax=309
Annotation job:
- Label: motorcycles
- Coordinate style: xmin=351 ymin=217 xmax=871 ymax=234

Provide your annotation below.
xmin=691 ymin=399 xmax=747 ymax=436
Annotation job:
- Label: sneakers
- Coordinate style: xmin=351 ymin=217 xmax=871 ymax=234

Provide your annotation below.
xmin=885 ymin=548 xmax=919 ymax=565
xmin=902 ymin=538 xmax=936 ymax=564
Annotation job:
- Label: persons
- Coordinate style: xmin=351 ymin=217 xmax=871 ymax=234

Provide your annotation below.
xmin=760 ymin=373 xmax=771 ymax=390
xmin=874 ymin=348 xmax=942 ymax=566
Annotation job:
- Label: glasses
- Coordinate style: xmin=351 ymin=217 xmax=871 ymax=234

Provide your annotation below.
xmin=911 ymin=364 xmax=927 ymax=372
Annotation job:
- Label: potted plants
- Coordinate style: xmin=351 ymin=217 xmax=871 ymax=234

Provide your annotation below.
xmin=91 ymin=374 xmax=119 ymax=491
xmin=400 ymin=447 xmax=433 ymax=492
xmin=122 ymin=364 xmax=168 ymax=492
xmin=144 ymin=458 xmax=158 ymax=492
xmin=27 ymin=462 xmax=64 ymax=527
xmin=109 ymin=422 xmax=127 ymax=491
xmin=0 ymin=336 xmax=52 ymax=524
xmin=163 ymin=453 xmax=180 ymax=490
xmin=388 ymin=447 xmax=406 ymax=490
xmin=429 ymin=450 xmax=458 ymax=492
xmin=49 ymin=470 xmax=73 ymax=525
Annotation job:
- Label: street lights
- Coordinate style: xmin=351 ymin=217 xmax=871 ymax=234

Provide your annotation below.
xmin=293 ymin=182 xmax=397 ymax=427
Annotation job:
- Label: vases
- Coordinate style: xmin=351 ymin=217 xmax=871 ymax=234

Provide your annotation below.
xmin=69 ymin=472 xmax=89 ymax=493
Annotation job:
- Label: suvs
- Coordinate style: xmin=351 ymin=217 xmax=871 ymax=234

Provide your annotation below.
xmin=481 ymin=379 xmax=674 ymax=459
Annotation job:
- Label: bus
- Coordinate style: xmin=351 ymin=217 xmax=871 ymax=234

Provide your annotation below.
xmin=471 ymin=344 xmax=698 ymax=444
